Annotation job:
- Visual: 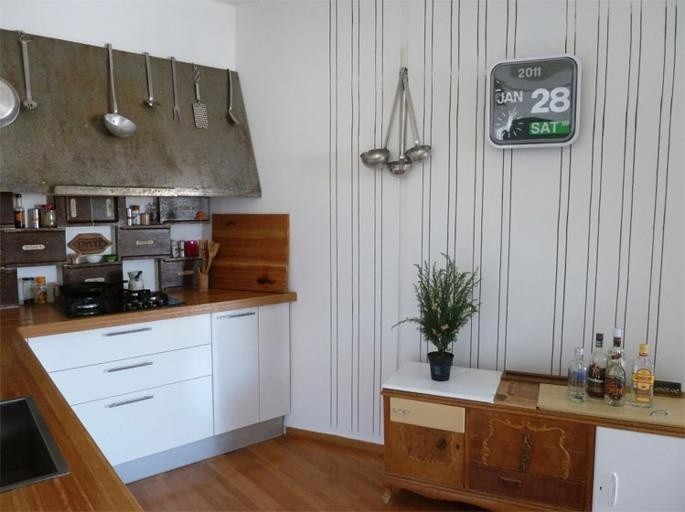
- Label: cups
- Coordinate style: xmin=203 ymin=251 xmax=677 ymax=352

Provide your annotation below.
xmin=71 ymin=257 xmax=81 ymax=263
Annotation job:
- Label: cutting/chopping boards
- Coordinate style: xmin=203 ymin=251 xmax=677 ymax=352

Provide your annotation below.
xmin=210 ymin=213 xmax=289 ymax=293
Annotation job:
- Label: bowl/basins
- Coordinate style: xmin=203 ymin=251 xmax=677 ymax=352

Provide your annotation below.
xmin=87 ymin=254 xmax=102 ymax=264
xmin=103 ymin=254 xmax=118 ymax=262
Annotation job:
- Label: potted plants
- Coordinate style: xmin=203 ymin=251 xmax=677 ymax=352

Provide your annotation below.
xmin=389 ymin=253 xmax=484 ymax=381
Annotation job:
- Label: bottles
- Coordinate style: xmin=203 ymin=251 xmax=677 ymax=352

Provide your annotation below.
xmin=628 ymin=343 xmax=655 ymax=409
xmin=588 ymin=333 xmax=609 ymax=398
xmin=604 ymin=350 xmax=626 ymax=408
xmin=127 ymin=205 xmax=159 ymax=226
xmin=22 ymin=276 xmax=47 ymax=306
xmin=567 ymin=349 xmax=589 ymax=403
xmin=13 ymin=194 xmax=27 ymax=228
xmin=608 ymin=328 xmax=625 ymax=356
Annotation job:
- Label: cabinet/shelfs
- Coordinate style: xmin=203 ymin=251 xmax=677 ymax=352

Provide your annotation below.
xmin=592 ymin=414 xmax=685 ymax=512
xmin=465 ymin=398 xmax=595 ymax=512
xmin=211 ymin=289 xmax=299 ymax=453
xmin=7 ymin=302 xmax=215 ymax=484
xmin=0 ymin=192 xmax=211 ymax=303
xmin=380 ymin=389 xmax=465 ymax=503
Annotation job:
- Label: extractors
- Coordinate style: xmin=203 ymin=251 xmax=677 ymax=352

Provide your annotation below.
xmin=52 ymin=185 xmax=178 ymax=198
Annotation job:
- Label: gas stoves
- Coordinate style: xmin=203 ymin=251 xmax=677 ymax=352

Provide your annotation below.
xmin=53 ymin=287 xmax=183 ymax=317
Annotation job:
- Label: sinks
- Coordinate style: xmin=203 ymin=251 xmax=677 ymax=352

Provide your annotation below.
xmin=0 ymin=395 xmax=71 ymax=494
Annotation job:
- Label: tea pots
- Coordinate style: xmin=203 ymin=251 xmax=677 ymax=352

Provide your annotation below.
xmin=125 ymin=270 xmax=145 ymax=291
